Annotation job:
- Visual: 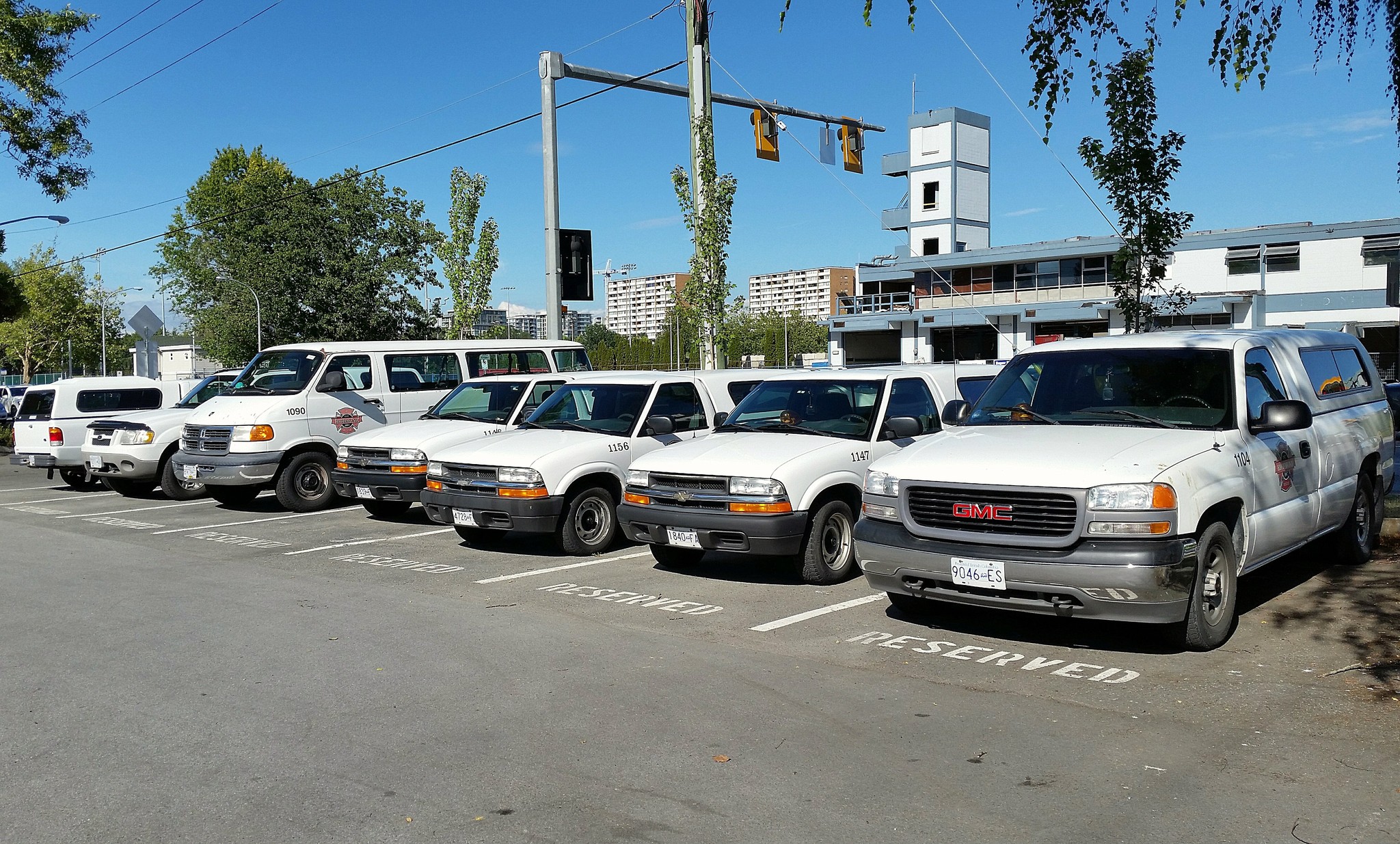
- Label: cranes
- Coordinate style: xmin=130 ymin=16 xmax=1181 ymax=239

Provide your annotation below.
xmin=593 ymin=258 xmax=628 ymax=329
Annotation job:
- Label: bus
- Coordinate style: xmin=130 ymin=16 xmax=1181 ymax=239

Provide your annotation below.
xmin=169 ymin=337 xmax=597 ymax=514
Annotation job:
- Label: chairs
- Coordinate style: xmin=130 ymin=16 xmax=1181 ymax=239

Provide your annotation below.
xmin=542 ymin=390 xmax=564 ymax=416
xmin=813 ymin=393 xmax=852 ymax=419
xmin=668 ymin=395 xmax=697 ymax=415
xmin=326 ymin=365 xmax=347 ymax=389
xmin=617 ymin=394 xmax=641 ymax=421
xmin=1070 ymin=373 xmax=1130 ymax=410
xmin=886 ymin=392 xmax=922 ymax=419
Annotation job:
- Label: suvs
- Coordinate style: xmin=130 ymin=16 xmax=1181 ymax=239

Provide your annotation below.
xmin=613 ymin=358 xmax=1014 ymax=586
xmin=849 ymin=322 xmax=1394 ymax=651
xmin=8 ymin=375 xmax=232 ymax=492
xmin=329 ymin=366 xmax=667 ymax=522
xmin=79 ymin=364 xmax=426 ymax=503
xmin=418 ymin=363 xmax=815 ymax=554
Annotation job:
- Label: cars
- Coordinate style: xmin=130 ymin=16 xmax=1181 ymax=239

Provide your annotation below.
xmin=0 ymin=384 xmax=34 ymax=417
xmin=0 ymin=397 xmax=8 ymax=424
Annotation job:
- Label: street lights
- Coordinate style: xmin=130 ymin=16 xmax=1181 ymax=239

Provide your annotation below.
xmin=500 ymin=286 xmax=515 ymax=339
xmin=215 ymin=275 xmax=262 ymax=353
xmin=103 ymin=287 xmax=143 ymax=377
xmin=620 ymin=262 xmax=637 ymax=347
